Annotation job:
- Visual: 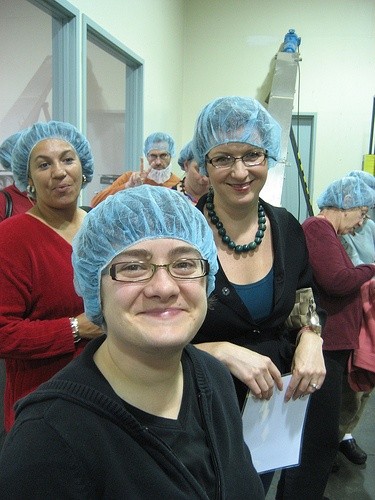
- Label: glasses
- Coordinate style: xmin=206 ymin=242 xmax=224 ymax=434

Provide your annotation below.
xmin=148 ymin=154 xmax=171 ymax=159
xmin=358 ymin=206 xmax=367 ymax=219
xmin=103 ymin=259 xmax=210 ymax=283
xmin=206 ymin=152 xmax=268 ymax=169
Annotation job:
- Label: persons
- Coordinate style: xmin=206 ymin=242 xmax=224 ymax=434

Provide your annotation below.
xmin=295 ymin=176 xmax=375 ymax=472
xmin=89 ymin=131 xmax=181 ymax=209
xmin=168 ymin=140 xmax=211 ymax=207
xmin=0 ymin=120 xmax=103 ymax=430
xmin=333 ymin=171 xmax=374 ymax=463
xmin=0 ymin=130 xmax=37 ymax=222
xmin=188 ymin=97 xmax=327 ymax=492
xmin=0 ymin=183 xmax=266 ymax=500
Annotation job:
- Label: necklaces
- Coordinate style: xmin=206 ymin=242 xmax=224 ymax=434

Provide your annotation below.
xmin=180 ymin=176 xmax=198 ymax=203
xmin=206 ymin=186 xmax=267 ymax=253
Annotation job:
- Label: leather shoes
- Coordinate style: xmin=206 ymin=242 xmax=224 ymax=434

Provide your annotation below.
xmin=341 ymin=438 xmax=368 ymax=464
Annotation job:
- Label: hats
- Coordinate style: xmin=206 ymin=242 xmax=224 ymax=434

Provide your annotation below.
xmin=345 ymin=170 xmax=375 ymax=190
xmin=0 ymin=133 xmax=19 ymax=172
xmin=318 ymin=177 xmax=375 ymax=209
xmin=144 ymin=133 xmax=175 ymax=158
xmin=177 ymin=141 xmax=193 ymax=170
xmin=71 ymin=183 xmax=218 ymax=334
xmin=192 ymin=96 xmax=282 ymax=177
xmin=12 ymin=121 xmax=94 ymax=196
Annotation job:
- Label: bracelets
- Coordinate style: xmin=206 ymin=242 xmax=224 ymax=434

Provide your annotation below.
xmin=69 ymin=317 xmax=81 ymax=344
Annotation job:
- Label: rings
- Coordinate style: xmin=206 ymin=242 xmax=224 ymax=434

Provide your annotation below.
xmin=309 ymin=384 xmax=317 ymax=388
